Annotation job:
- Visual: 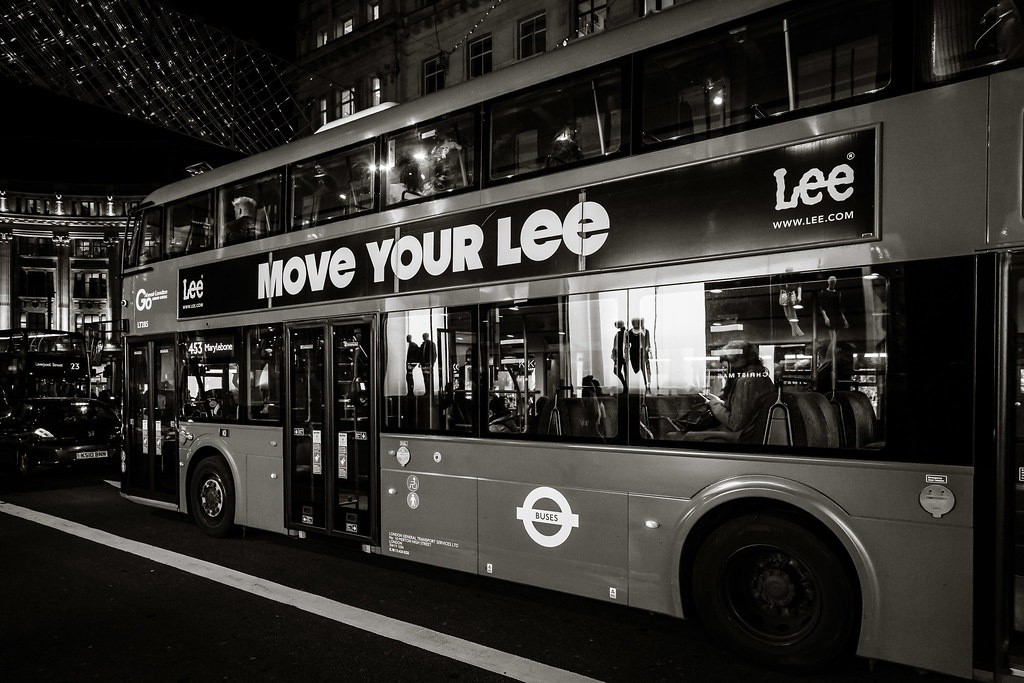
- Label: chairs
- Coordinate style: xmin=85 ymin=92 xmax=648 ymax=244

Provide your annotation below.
xmin=798 ymin=389 xmax=880 ymax=450
xmin=536 ymin=392 xmax=779 ymax=441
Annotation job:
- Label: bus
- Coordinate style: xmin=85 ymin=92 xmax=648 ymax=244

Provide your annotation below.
xmin=0 ymin=328 xmax=91 ymax=407
xmin=116 ymin=0 xmax=1023 ymax=682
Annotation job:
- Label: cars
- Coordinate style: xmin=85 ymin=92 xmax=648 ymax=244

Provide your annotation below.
xmin=0 ymin=399 xmax=126 ymax=491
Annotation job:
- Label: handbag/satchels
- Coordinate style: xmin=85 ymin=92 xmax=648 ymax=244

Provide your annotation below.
xmin=676 ymin=410 xmax=721 ymax=434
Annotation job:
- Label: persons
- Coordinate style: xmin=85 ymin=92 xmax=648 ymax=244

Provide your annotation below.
xmin=813 ymin=346 xmax=832 ymax=391
xmin=820 ymin=277 xmax=848 ymax=360
xmin=406 ymin=333 xmax=436 ymax=397
xmin=612 ymin=318 xmax=653 ymax=391
xmin=224 ymin=197 xmax=256 ymax=242
xmin=490 ymin=397 xmax=517 ymax=431
xmin=528 ymin=397 xmax=548 ymax=431
xmin=780 ymin=268 xmax=805 ymax=336
xmin=705 ymin=340 xmax=775 ymax=432
